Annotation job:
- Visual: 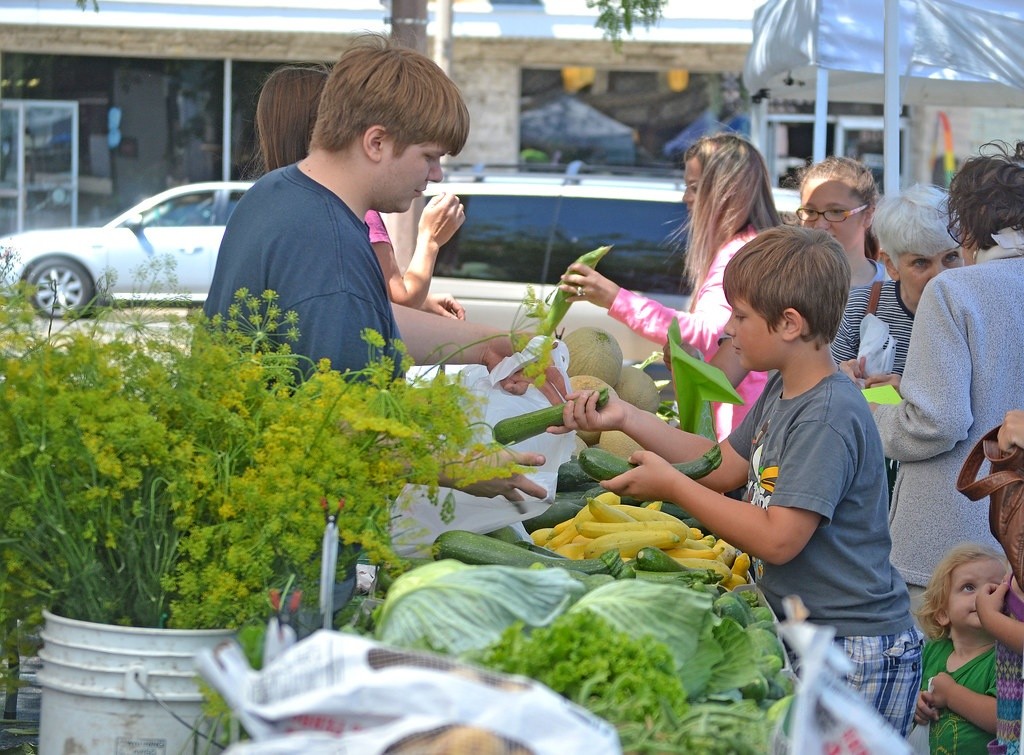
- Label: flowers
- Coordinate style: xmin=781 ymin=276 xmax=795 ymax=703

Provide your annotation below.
xmin=0 ymin=251 xmax=553 ymax=702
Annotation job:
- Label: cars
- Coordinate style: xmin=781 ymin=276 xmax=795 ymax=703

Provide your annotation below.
xmin=1 ymin=181 xmax=259 ymax=319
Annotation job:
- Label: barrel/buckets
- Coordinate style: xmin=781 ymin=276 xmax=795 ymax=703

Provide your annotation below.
xmin=38 ymin=607 xmax=244 ymax=755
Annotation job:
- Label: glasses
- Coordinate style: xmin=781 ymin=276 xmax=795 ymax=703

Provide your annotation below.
xmin=946 ymin=203 xmax=982 ymax=245
xmin=795 ymin=203 xmax=868 ymax=222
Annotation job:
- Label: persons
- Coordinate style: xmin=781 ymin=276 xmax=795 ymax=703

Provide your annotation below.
xmin=915 ymin=541 xmax=1011 ymax=755
xmin=546 ymin=226 xmax=923 ymax=741
xmin=975 ymin=409 xmax=1024 ymax=755
xmin=797 ymin=138 xmax=1024 ymax=636
xmin=202 ymin=32 xmax=567 ymax=642
xmin=558 ymin=133 xmax=787 ymax=446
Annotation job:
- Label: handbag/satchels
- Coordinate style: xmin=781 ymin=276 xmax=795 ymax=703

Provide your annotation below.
xmin=956 ymin=422 xmax=1024 ymax=592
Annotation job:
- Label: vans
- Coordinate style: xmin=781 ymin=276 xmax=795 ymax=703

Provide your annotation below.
xmin=386 ymin=177 xmax=800 ymax=367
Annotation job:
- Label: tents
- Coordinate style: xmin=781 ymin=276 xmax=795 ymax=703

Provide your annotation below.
xmin=743 ymin=1 xmax=1024 ymax=200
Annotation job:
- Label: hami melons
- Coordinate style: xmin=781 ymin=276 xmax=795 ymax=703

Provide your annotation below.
xmin=558 ymin=325 xmax=660 ymax=461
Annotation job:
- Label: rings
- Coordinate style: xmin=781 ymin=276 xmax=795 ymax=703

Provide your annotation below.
xmin=577 ymin=287 xmax=583 ymax=296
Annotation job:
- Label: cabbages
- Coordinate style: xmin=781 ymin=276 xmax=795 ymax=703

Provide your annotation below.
xmin=564 ymin=580 xmax=772 ymax=755
xmin=374 ymin=558 xmax=584 ymax=667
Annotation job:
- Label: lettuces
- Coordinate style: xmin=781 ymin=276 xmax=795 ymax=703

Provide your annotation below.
xmin=414 ymin=605 xmax=714 ymax=755
